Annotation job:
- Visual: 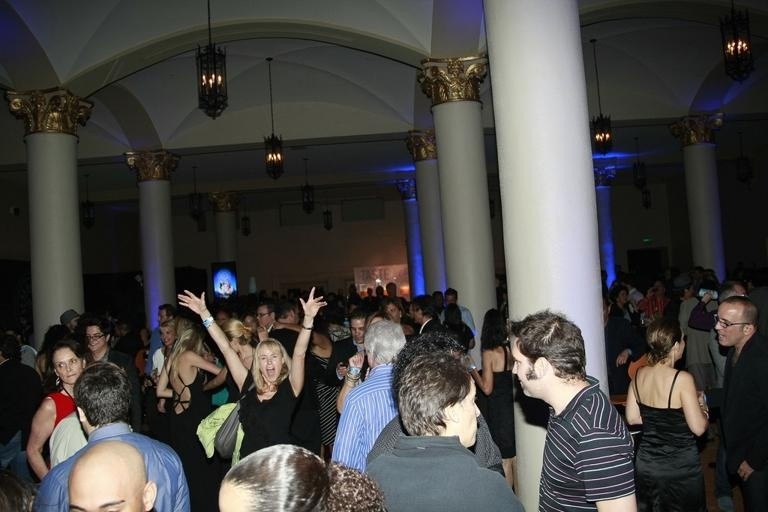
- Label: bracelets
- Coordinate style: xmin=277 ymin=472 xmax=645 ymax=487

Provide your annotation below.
xmin=699 ymin=404 xmax=709 ymax=420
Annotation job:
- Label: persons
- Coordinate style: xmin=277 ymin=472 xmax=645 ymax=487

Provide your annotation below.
xmin=1 ymin=272 xmax=525 ymax=511
xmin=511 ymin=314 xmax=637 ymax=512
xmin=625 ymin=315 xmax=709 ymax=512
xmin=602 ymin=267 xmax=764 ymax=391
xmin=704 ymin=297 xmax=768 ymax=512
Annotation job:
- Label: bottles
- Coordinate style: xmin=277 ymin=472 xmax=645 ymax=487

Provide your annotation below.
xmin=344 ymin=318 xmax=349 ymax=328
xmin=699 ymin=289 xmax=720 ymax=300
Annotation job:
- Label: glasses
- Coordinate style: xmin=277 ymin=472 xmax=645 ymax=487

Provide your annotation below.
xmin=255 ymin=313 xmax=271 ymax=317
xmin=85 ymin=334 xmax=106 ymax=340
xmin=713 ymin=313 xmax=749 ymax=328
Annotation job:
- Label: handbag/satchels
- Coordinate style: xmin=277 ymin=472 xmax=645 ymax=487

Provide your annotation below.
xmin=213 ymin=401 xmax=241 ymax=460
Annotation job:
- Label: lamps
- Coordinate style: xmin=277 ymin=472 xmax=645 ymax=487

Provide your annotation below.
xmin=300 ymin=157 xmax=314 ymax=215
xmin=81 ymin=174 xmax=95 ymax=227
xmin=196 ymin=0 xmax=229 ymax=120
xmin=188 ymin=166 xmax=202 ymax=221
xmin=717 ymin=0 xmax=756 ymax=85
xmin=589 ymin=37 xmax=613 ymax=156
xmin=322 ymin=190 xmax=333 ymax=231
xmin=263 ymin=57 xmax=284 ymax=181
xmin=240 ymin=196 xmax=251 ymax=237
